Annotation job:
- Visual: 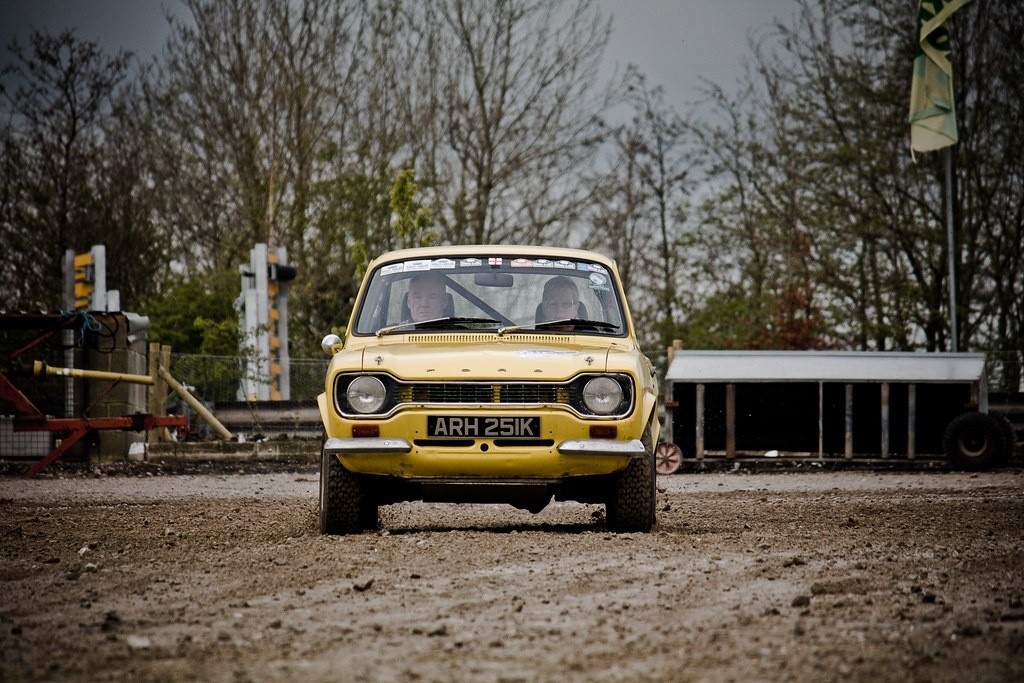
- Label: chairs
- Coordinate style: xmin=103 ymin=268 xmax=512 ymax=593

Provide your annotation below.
xmin=533 ymin=302 xmax=591 ymax=332
xmin=399 ymin=291 xmax=455 ymax=323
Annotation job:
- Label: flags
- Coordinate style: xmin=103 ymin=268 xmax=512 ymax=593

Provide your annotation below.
xmin=909 ymin=0 xmax=969 ymax=164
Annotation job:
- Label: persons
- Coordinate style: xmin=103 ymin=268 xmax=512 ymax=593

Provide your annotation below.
xmin=543 ymin=276 xmax=597 ymax=330
xmin=407 ymin=273 xmax=449 ymax=325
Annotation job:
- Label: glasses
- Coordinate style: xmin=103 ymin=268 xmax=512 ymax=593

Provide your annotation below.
xmin=545 ymin=300 xmax=577 ymax=310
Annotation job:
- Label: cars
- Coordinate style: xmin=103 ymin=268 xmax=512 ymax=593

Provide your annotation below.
xmin=317 ymin=244 xmax=662 ymax=535
